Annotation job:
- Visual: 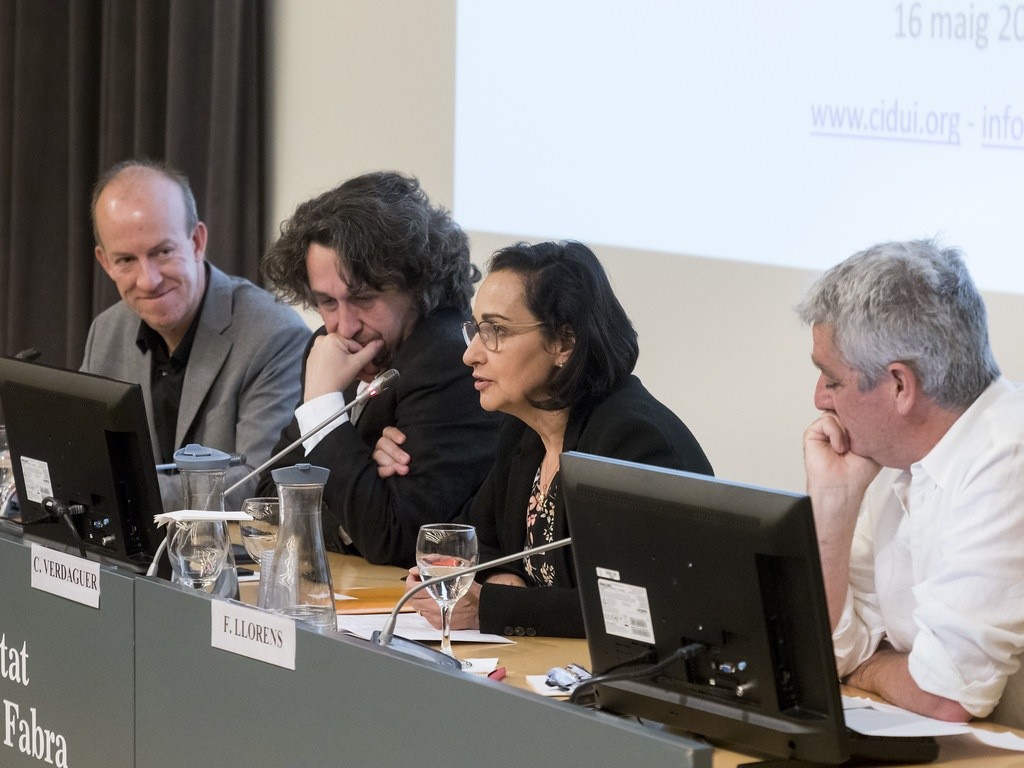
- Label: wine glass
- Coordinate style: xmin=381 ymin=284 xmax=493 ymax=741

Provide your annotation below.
xmin=1 ymin=427 xmax=17 ymax=520
xmin=417 ymin=525 xmax=479 ymax=668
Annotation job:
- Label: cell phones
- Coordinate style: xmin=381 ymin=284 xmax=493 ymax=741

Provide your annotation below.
xmin=187 ymin=567 xmax=254 ymax=576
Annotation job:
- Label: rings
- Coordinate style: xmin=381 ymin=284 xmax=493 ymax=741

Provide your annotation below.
xmin=419 ymin=610 xmax=423 ymax=617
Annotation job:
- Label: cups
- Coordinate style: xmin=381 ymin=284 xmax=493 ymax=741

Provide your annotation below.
xmin=257 ymin=551 xmax=301 ymax=611
xmin=239 ymin=496 xmax=282 ymax=563
xmin=167 ymin=520 xmax=229 ymax=590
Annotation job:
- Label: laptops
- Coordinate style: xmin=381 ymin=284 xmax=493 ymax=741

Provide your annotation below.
xmin=559 ymin=449 xmax=939 ymax=764
xmin=0 ymin=354 xmax=259 ymax=572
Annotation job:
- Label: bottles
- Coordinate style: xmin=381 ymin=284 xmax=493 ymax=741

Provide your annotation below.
xmin=264 ymin=464 xmax=338 ymax=633
xmin=174 ymin=445 xmax=241 ymax=602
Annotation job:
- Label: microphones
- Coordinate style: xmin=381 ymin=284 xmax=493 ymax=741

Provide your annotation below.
xmin=0 ymin=454 xmax=246 ymax=518
xmin=14 ymin=346 xmax=43 ymax=361
xmin=40 ymin=496 xmax=88 ymax=559
xmin=146 ymin=368 xmax=399 ymax=577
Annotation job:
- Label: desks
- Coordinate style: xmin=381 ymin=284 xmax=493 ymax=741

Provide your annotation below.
xmin=0 ymin=498 xmax=1024 ymax=768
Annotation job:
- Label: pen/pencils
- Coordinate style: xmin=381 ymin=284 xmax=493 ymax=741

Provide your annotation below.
xmin=487 ymin=667 xmax=506 ymax=682
xmin=401 ymin=560 xmax=461 ymax=582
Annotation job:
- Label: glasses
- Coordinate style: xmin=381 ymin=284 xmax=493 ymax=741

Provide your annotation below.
xmin=460 ymin=321 xmax=546 ymax=352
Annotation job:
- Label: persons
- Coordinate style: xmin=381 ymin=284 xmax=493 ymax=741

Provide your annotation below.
xmin=79 ymin=159 xmax=312 ymax=520
xmin=406 ymin=238 xmax=713 ymax=638
xmin=260 ymin=170 xmax=505 ymax=575
xmin=796 ymin=238 xmax=1024 ymax=726
